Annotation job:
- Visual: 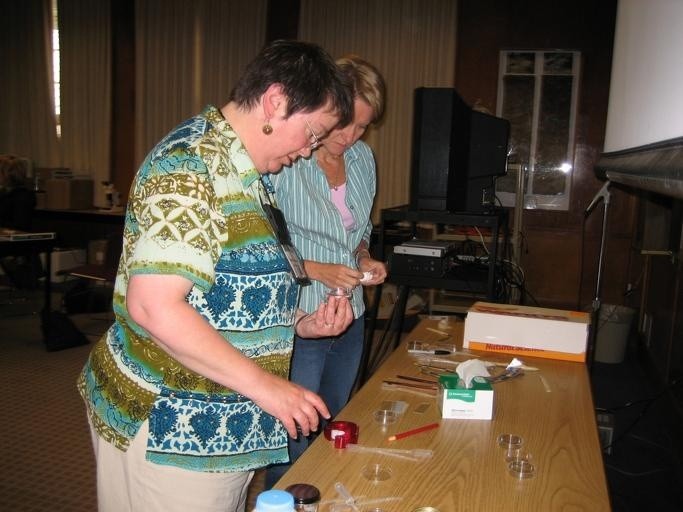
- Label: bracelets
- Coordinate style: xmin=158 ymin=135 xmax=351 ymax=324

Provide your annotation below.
xmin=354 ymin=247 xmax=370 ymax=270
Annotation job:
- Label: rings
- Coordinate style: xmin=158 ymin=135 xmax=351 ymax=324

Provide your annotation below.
xmin=325 ymin=322 xmax=334 ymax=327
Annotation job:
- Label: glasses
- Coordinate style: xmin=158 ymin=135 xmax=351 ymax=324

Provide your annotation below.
xmin=289 ymin=94 xmax=325 ymax=151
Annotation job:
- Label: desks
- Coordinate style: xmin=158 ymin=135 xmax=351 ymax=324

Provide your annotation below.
xmin=0 ymin=205 xmax=121 ymax=316
xmin=246 ymin=312 xmax=611 ymax=512
xmin=373 ymin=202 xmax=511 ymax=351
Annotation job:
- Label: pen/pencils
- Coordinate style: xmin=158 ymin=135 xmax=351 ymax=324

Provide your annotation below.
xmin=427 ymin=328 xmax=448 ymax=336
xmin=381 ymin=350 xmax=540 ymax=399
xmin=388 ymin=423 xmax=440 ymax=441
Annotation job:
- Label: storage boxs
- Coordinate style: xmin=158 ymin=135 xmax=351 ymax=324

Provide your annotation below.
xmin=45 ymin=177 xmax=93 ymax=211
xmin=461 ymin=298 xmax=594 ymax=365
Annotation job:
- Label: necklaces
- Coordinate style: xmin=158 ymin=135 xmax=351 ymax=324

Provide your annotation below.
xmin=320 ymin=164 xmax=344 ymax=191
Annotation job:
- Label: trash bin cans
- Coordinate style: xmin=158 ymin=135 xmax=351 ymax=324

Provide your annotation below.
xmin=593 ymin=304 xmax=636 ymax=364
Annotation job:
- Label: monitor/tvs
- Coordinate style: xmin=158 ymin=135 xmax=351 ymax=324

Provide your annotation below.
xmin=409 ymin=86 xmax=511 ymax=216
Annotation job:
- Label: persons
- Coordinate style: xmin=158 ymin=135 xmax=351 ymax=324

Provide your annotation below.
xmin=260 ymin=53 xmax=390 ymax=491
xmin=75 ymin=40 xmax=356 ymax=512
